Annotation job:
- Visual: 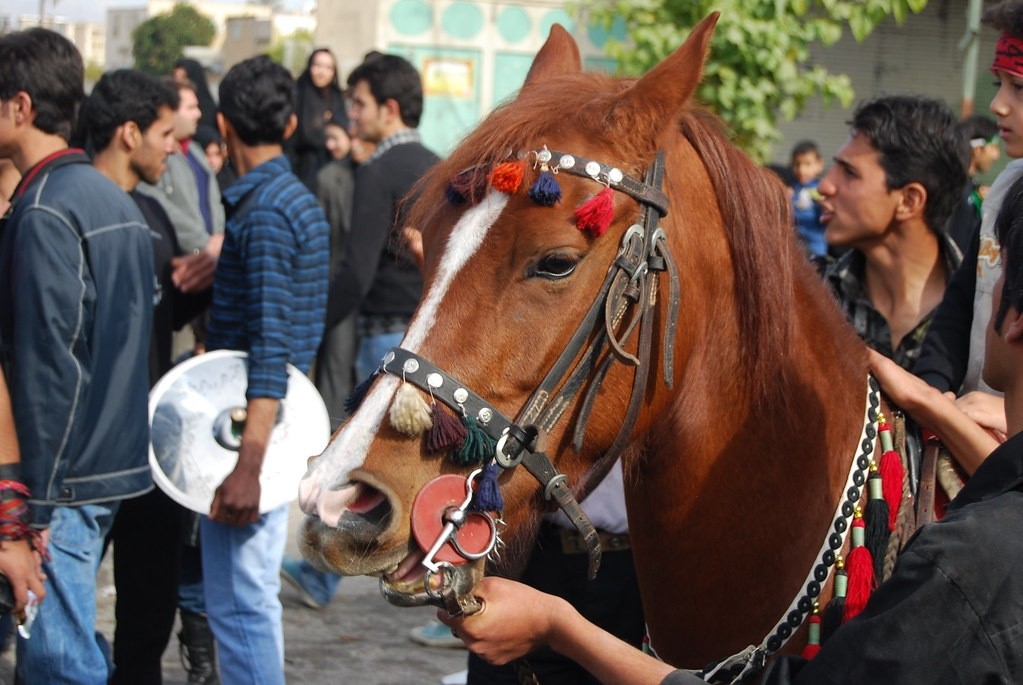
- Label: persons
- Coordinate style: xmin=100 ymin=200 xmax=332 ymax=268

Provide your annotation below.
xmin=818 ymin=94 xmax=977 ymax=431
xmin=1 ymin=368 xmax=44 ymax=613
xmin=460 ymin=173 xmax=1023 ymax=685
xmin=63 ymin=66 xmax=215 ymax=682
xmin=1 ymin=26 xmax=161 ymax=685
xmin=985 ymin=0 xmax=1023 ymax=155
xmin=955 ymin=114 xmax=1005 ymax=218
xmin=781 ymin=141 xmax=836 ymax=256
xmin=79 ymin=43 xmax=371 ymax=421
xmin=192 ymin=55 xmax=329 ymax=683
xmin=281 ymin=48 xmax=473 ymax=659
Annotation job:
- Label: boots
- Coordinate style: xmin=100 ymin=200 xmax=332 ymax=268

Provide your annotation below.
xmin=176 ymin=604 xmax=220 ymax=684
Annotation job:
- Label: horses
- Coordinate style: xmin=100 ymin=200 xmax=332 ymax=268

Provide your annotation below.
xmin=295 ymin=10 xmax=1009 ymax=685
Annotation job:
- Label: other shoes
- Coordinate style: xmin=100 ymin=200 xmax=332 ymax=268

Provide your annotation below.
xmin=280 ymin=557 xmax=343 ymax=607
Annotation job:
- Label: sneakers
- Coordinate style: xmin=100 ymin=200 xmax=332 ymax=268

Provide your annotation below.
xmin=409 ymin=619 xmax=469 ymax=650
